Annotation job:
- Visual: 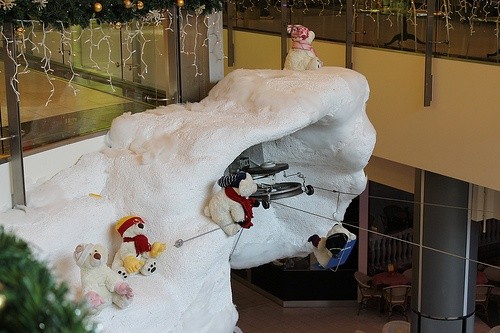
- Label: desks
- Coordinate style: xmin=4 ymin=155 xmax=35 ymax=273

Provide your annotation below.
xmin=373 ymin=272 xmax=409 ymax=312
xmin=358 ymin=8 xmax=449 ymax=49
xmin=486 ymin=47 xmax=500 ymax=58
xmin=477 ymin=271 xmax=488 ymax=285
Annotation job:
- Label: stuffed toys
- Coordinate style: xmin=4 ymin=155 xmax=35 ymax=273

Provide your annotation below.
xmin=203 ymin=171 xmax=258 ymax=237
xmin=307 ymin=223 xmax=356 ymax=268
xmin=285 ymin=24 xmax=321 ymax=71
xmin=73 ymin=243 xmax=134 ymax=316
xmin=111 ymin=214 xmax=166 ymax=278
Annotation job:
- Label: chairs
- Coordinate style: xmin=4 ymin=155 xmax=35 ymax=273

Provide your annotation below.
xmin=489 ymin=286 xmax=500 ymax=295
xmin=403 ymin=268 xmax=413 ymax=282
xmin=353 ymin=271 xmax=381 ymax=316
xmin=489 ymin=326 xmax=500 ymax=333
xmin=383 ymin=282 xmax=413 ymax=322
xmin=484 ymin=267 xmax=500 ymax=283
xmin=383 ymin=205 xmax=410 ymax=229
xmin=475 ymin=284 xmax=495 ymax=323
xmin=382 ymin=320 xmax=410 ymax=333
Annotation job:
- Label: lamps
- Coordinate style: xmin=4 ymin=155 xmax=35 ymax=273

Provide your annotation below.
xmin=387 ymin=264 xmax=394 ymax=276
